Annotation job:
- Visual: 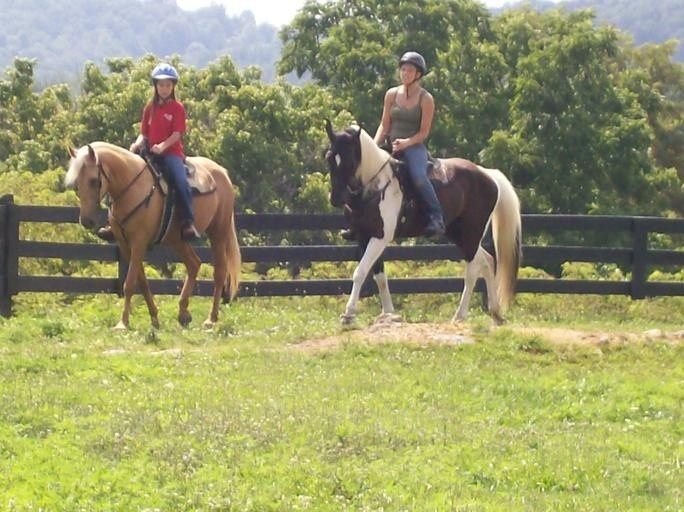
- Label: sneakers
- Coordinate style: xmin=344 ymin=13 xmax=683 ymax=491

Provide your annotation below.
xmin=183 ymin=224 xmax=202 ymax=241
xmin=98 ymin=226 xmax=114 ymax=240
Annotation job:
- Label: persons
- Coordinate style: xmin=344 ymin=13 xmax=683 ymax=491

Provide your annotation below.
xmin=339 ymin=51 xmax=445 ymax=240
xmin=93 ymin=62 xmax=199 ymax=240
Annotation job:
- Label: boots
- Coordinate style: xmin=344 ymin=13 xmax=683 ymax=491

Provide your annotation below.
xmin=340 ymin=229 xmax=356 ymax=241
xmin=413 ymin=177 xmax=446 ymax=236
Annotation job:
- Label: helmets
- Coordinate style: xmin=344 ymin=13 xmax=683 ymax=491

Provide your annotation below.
xmin=399 ymin=50 xmax=426 ymax=70
xmin=150 ymin=63 xmax=179 ymax=80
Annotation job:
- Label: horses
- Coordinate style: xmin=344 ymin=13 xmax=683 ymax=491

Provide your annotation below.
xmin=62 ymin=141 xmax=242 ymax=345
xmin=322 ymin=117 xmax=523 ymax=331
